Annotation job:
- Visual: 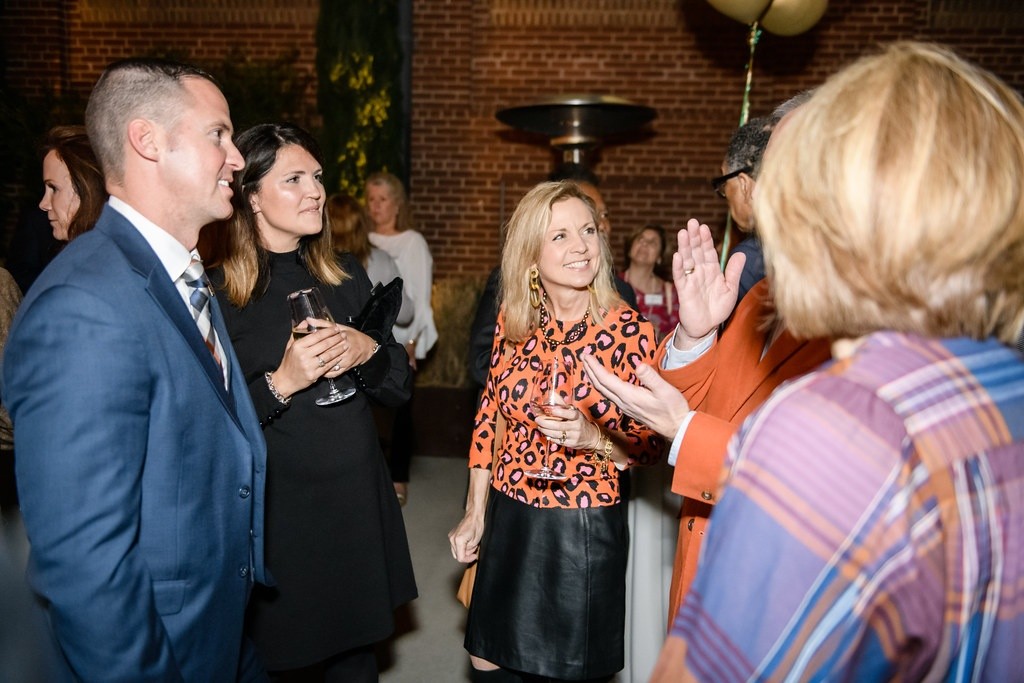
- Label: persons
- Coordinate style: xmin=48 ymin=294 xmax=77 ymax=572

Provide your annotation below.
xmin=581 ymin=220 xmax=849 ymax=637
xmin=711 ymin=116 xmax=775 ymax=330
xmin=361 ymin=173 xmax=439 ymax=506
xmin=0 ymin=53 xmax=269 ymax=683
xmin=324 ymin=193 xmax=414 ymax=327
xmin=0 ymin=266 xmax=23 ymax=519
xmin=618 ymin=220 xmax=678 ymax=344
xmin=40 ymin=125 xmax=109 ymax=241
xmin=646 ymin=40 xmax=1024 ymax=683
xmin=448 ymin=160 xmax=658 ymax=683
xmin=205 ymin=122 xmax=419 ymax=683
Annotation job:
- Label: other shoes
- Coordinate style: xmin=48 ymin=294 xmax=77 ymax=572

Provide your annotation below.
xmin=396 ymin=490 xmax=408 ymax=507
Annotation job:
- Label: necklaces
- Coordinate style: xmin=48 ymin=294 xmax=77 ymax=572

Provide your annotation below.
xmin=540 ymin=293 xmax=590 ymax=344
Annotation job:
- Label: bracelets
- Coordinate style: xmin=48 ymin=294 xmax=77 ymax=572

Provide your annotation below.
xmin=595 ymin=434 xmax=613 ymax=462
xmin=582 ymin=421 xmax=601 ymax=454
xmin=409 ymin=340 xmax=414 ymax=344
xmin=267 ymin=373 xmax=286 ymax=403
xmin=373 ymin=344 xmax=378 ymax=351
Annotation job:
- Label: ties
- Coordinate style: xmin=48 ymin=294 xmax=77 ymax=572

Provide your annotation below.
xmin=182 ymin=261 xmax=221 ymax=367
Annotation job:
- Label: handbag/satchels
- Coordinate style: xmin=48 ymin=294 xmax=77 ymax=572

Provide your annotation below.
xmin=371 ymin=334 xmax=413 ymax=413
xmin=347 ymin=272 xmax=403 ymax=394
xmin=455 ymin=547 xmax=479 ymax=610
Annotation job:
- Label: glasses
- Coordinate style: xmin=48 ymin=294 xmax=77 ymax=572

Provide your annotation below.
xmin=712 ymin=166 xmax=751 ymax=198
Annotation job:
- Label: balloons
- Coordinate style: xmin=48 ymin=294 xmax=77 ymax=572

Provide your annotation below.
xmin=707 ymin=0 xmax=829 ymax=37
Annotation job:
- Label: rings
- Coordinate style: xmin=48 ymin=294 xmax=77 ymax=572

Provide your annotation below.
xmin=335 ymin=364 xmax=340 ymax=370
xmin=318 ymin=357 xmax=324 ymax=367
xmin=685 ymin=268 xmax=694 ymax=274
xmin=563 ymin=432 xmax=566 ymax=440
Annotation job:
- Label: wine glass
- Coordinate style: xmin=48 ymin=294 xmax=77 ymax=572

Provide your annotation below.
xmin=287 ymin=286 xmax=357 ymax=406
